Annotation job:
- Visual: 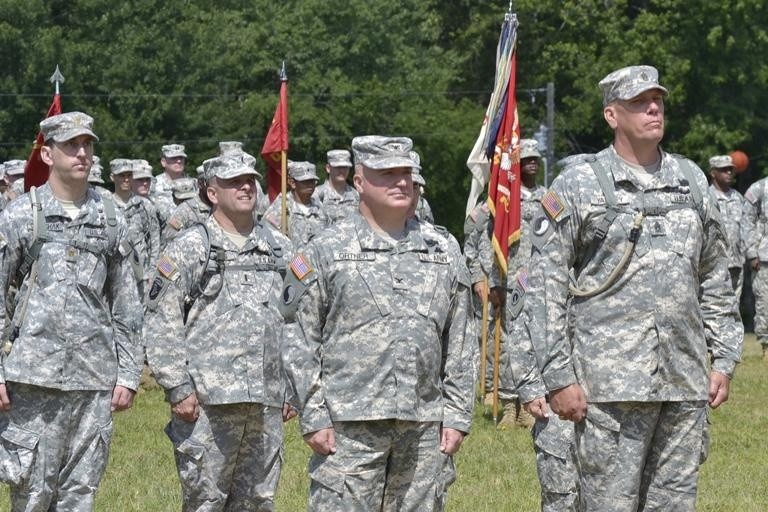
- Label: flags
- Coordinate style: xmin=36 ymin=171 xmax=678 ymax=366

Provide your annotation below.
xmin=464 ymin=13 xmax=522 ymax=279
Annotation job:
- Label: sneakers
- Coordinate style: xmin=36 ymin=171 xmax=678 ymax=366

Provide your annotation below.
xmin=483 ymin=390 xmax=495 ymax=407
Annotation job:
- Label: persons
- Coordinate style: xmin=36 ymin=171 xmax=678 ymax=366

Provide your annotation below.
xmin=1 ymin=111 xmax=479 ymax=511
xmin=467 ymin=66 xmax=767 ymax=512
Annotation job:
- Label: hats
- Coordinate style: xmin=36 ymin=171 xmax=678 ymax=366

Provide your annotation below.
xmin=707 ymin=155 xmax=734 ymax=171
xmin=218 ymin=141 xmax=243 ymax=156
xmin=161 ymin=143 xmax=187 ymax=158
xmin=4 ymin=160 xmax=26 ymax=175
xmin=596 ymin=64 xmax=668 ymax=108
xmin=131 ymin=165 xmax=154 ymax=181
xmin=289 ymin=162 xmax=319 ymax=180
xmin=202 ymin=156 xmax=263 ymax=181
xmin=517 ymin=138 xmax=541 ymax=158
xmin=350 ymin=134 xmax=422 ymax=170
xmin=325 ymin=149 xmax=353 ymax=167
xmin=39 ymin=111 xmax=99 ymax=144
xmin=171 ymin=177 xmax=197 ymax=199
xmin=196 ymin=165 xmax=205 ymax=181
xmin=130 ymin=159 xmax=148 ymax=165
xmin=109 ymin=158 xmax=134 ymax=174
xmin=407 ymin=151 xmax=425 ymax=185
xmin=226 ymin=150 xmax=256 ymax=168
xmin=0 ymin=163 xmax=6 ymax=181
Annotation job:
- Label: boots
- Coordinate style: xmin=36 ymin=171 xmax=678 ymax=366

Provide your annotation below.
xmin=495 ymin=397 xmax=517 ymax=432
xmin=516 ymin=400 xmax=536 ymax=428
